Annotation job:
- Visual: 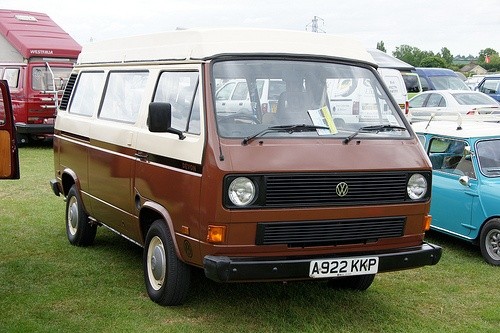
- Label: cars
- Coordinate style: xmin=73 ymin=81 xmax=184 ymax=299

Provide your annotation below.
xmin=379 ymin=66 xmax=500 ymax=266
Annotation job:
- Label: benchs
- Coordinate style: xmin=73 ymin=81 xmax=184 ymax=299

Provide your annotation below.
xmin=428 ymin=152 xmax=465 ymax=170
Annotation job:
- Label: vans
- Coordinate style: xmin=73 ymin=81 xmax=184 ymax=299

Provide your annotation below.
xmin=50 ymin=50 xmax=446 ymax=306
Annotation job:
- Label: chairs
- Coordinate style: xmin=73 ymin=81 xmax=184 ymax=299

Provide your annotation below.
xmin=276 ymin=90 xmax=317 ymax=126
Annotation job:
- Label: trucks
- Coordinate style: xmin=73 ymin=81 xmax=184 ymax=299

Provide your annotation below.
xmin=0 ymin=9 xmax=83 ymax=146
